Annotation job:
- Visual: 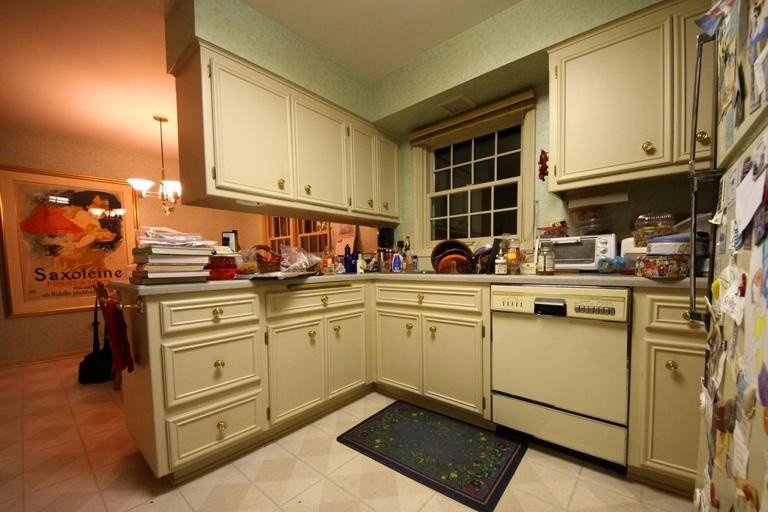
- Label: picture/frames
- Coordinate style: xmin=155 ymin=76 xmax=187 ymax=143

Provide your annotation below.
xmin=0 ymin=163 xmax=141 ymax=320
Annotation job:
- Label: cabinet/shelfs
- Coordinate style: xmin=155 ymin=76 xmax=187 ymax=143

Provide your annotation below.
xmin=373 ymin=281 xmax=497 ymax=433
xmin=175 ymin=45 xmax=349 ymax=226
xmin=262 ymin=279 xmax=372 ymax=447
xmin=626 ymin=288 xmax=708 ymax=503
xmin=545 ymin=2 xmax=719 ymax=201
xmin=116 ymin=287 xmax=269 ymax=491
xmin=347 ymin=112 xmax=401 ymax=231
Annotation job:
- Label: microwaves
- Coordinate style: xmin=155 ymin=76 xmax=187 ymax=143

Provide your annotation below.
xmin=535 ymin=233 xmax=617 ymax=270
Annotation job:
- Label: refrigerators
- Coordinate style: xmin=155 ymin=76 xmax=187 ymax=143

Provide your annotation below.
xmin=687 ymin=0 xmax=768 ymax=512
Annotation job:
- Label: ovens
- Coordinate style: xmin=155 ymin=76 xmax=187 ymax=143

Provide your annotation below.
xmin=489 ymin=284 xmax=635 ymax=474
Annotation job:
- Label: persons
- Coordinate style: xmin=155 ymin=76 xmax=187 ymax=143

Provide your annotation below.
xmin=17 ymin=192 xmax=112 ymax=259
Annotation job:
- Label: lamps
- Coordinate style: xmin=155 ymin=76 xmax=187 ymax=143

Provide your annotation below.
xmin=125 ymin=115 xmax=183 ymax=217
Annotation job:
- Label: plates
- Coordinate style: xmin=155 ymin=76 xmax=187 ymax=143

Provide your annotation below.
xmin=429 ymin=237 xmax=473 ymax=273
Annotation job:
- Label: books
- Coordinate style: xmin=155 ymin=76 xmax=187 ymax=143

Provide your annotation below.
xmin=128 ymin=244 xmax=214 ymax=284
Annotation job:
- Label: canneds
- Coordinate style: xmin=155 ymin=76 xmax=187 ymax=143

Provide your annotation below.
xmin=536 ymin=247 xmax=555 ymax=276
xmin=495 ymin=260 xmax=508 ymax=275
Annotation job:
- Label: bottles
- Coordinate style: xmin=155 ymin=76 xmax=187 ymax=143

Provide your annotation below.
xmin=506 ymin=237 xmax=523 ymax=274
xmin=494 ymin=248 xmax=508 ymax=275
xmin=321 ymin=246 xmax=335 ymax=275
xmin=342 ymin=235 xmax=419 ymax=273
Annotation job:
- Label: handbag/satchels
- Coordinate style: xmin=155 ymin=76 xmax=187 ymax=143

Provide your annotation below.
xmin=79 ymin=349 xmax=113 ymax=384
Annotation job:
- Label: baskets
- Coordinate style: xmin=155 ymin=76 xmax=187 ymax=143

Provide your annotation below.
xmin=256 ymin=245 xmax=280 ymax=272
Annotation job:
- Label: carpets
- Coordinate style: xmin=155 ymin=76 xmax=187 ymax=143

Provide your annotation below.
xmin=335 ymin=399 xmax=530 ymax=512
